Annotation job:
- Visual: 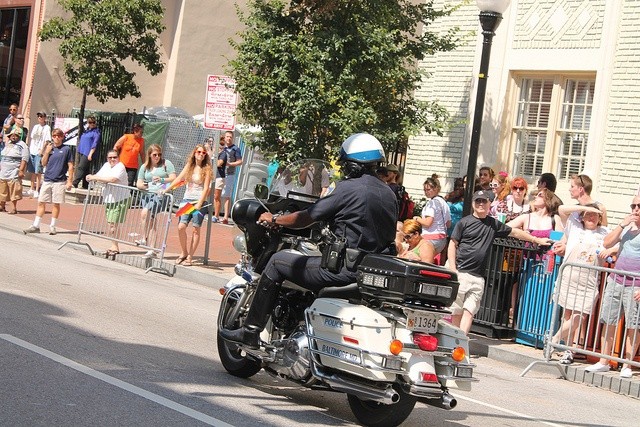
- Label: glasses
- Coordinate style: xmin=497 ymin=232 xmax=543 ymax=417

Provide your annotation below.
xmin=630 ymin=203 xmax=640 ymax=208
xmin=538 ymin=181 xmax=542 ymax=184
xmin=109 ymin=156 xmax=117 ymax=158
xmin=38 ymin=115 xmax=43 ymax=117
xmin=16 ymin=117 xmax=24 ymax=119
xmin=402 ymin=233 xmax=413 ymax=241
xmin=578 ymin=175 xmax=585 ymax=187
xmin=195 ymin=150 xmax=206 ymax=155
xmin=489 ymin=182 xmax=501 ymax=188
xmin=512 ymin=186 xmax=524 ymax=190
xmin=153 ymin=153 xmax=162 ymax=156
xmin=476 ymin=198 xmax=488 ymax=203
xmin=536 ymin=193 xmax=544 ymax=197
xmin=52 ymin=134 xmax=62 ymax=137
xmin=9 ymin=109 xmax=16 ymax=110
xmin=88 ymin=121 xmax=96 ymax=123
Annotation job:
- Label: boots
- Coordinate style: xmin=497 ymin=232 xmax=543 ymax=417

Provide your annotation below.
xmin=219 ymin=270 xmax=282 ymax=349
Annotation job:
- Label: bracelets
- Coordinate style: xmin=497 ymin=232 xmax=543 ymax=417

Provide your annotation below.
xmin=161 ymin=177 xmax=164 ymax=183
xmin=272 ymin=216 xmax=277 ymax=224
xmin=619 ymin=223 xmax=625 ymax=229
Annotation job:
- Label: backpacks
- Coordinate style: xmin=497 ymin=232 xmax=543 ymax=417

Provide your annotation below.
xmin=390 ymin=183 xmax=415 ymax=221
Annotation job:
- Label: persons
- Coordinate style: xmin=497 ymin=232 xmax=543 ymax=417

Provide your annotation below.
xmin=410 ymin=174 xmax=451 ymax=264
xmin=221 ymin=131 xmax=243 ymax=224
xmin=7 ymin=115 xmax=28 ymax=145
xmin=537 ymin=173 xmax=564 ymax=232
xmin=452 ymin=174 xmax=480 ymax=208
xmin=0 ymin=104 xmax=18 ymax=147
xmin=488 ymin=174 xmax=514 ymax=224
xmin=71 ymin=116 xmax=100 ymax=190
xmin=492 ymin=177 xmax=532 ymax=334
xmin=209 ymin=134 xmax=227 ymax=224
xmin=28 ymin=112 xmax=51 ymax=198
xmin=395 ymin=219 xmax=423 ymax=262
xmin=113 ymin=125 xmax=146 ymax=196
xmin=0 ymin=128 xmax=29 ymax=214
xmin=569 ymin=175 xmax=608 ymax=229
xmin=448 ymin=191 xmax=554 ymax=338
xmin=160 ymin=143 xmax=212 ymax=265
xmin=221 ymin=133 xmax=399 ymax=350
xmin=586 ymin=195 xmax=640 ymax=378
xmin=24 ymin=129 xmax=74 ymax=235
xmin=387 ymin=164 xmax=404 ymax=222
xmin=400 ymin=219 xmax=434 ymax=266
xmin=543 ymin=202 xmax=619 ymax=364
xmin=135 ymin=144 xmax=177 ymax=260
xmin=85 ymin=149 xmax=131 ymax=256
xmin=504 ymin=188 xmax=565 ymax=347
xmin=204 ymin=136 xmax=214 ymax=220
xmin=475 ymin=167 xmax=495 ymax=217
xmin=376 ymin=167 xmax=388 ymax=186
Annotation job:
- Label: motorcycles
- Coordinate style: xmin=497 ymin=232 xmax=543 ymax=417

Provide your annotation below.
xmin=216 ymin=158 xmax=480 ymax=426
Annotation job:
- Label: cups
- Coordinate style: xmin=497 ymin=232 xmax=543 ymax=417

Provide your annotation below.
xmin=497 ymin=213 xmax=506 ymax=225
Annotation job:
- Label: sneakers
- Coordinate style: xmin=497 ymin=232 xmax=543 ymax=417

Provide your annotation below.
xmin=212 ymin=216 xmax=221 ymax=223
xmin=23 ymin=224 xmax=40 ymax=233
xmin=543 ymin=334 xmax=555 ymax=361
xmin=560 ymin=349 xmax=576 ymax=364
xmin=25 ymin=190 xmax=36 ymax=196
xmin=143 ymin=250 xmax=158 ymax=258
xmin=620 ymin=367 xmax=634 ymax=377
xmin=556 ymin=342 xmax=588 ymax=358
xmin=222 ymin=218 xmax=228 ymax=224
xmin=50 ymin=225 xmax=57 ymax=234
xmin=134 ymin=238 xmax=148 ymax=246
xmin=34 ymin=191 xmax=39 ymax=198
xmin=584 ymin=361 xmax=611 ymax=371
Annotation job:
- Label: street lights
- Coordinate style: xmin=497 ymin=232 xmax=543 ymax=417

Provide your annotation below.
xmin=465 ymin=0 xmax=511 ymax=218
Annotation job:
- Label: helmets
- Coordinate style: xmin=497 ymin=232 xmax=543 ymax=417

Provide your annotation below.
xmin=335 ymin=132 xmax=387 ymax=165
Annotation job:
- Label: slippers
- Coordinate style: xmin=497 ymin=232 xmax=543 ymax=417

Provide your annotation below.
xmin=108 ymin=250 xmax=119 ymax=256
xmin=0 ymin=205 xmax=5 ymax=212
xmin=176 ymin=253 xmax=188 ymax=264
xmin=7 ymin=209 xmax=17 ymax=214
xmin=103 ymin=249 xmax=111 ymax=255
xmin=183 ymin=259 xmax=192 ymax=265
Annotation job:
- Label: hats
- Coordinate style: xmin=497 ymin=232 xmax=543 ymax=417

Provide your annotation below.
xmin=385 ymin=164 xmax=399 ymax=171
xmin=472 ymin=189 xmax=489 ymax=199
xmin=37 ymin=112 xmax=46 ymax=116
xmin=7 ymin=128 xmax=22 ymax=137
